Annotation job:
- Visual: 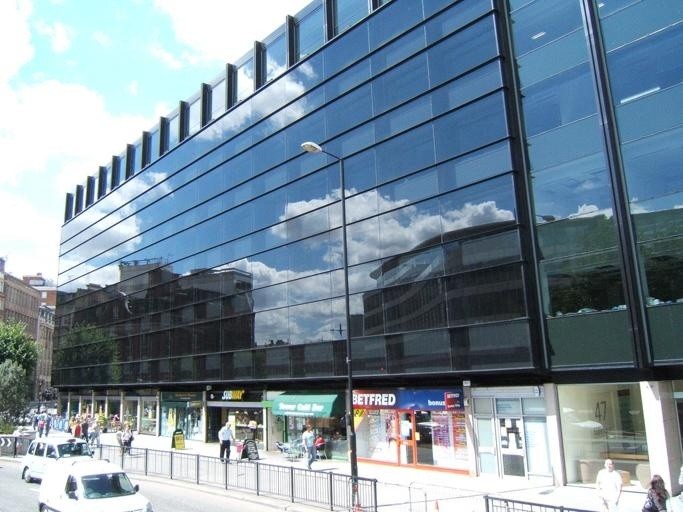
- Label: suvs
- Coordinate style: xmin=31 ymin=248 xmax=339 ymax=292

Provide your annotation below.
xmin=38 ymin=457 xmax=150 ymax=512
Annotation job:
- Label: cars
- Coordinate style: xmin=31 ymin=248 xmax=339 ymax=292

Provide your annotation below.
xmin=21 ymin=436 xmax=99 ymax=482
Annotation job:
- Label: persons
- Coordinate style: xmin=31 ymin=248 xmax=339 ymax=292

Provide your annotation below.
xmin=116 ymin=426 xmax=134 ymax=455
xmin=31 ymin=410 xmax=101 ymax=447
xmin=315 ymin=435 xmax=324 ymax=449
xmin=642 ymin=474 xmax=673 ymax=512
xmin=596 ymin=459 xmax=623 ymax=509
xmin=218 ymin=422 xmax=236 ymax=462
xmin=113 ymin=414 xmax=119 ymax=421
xmin=302 ymin=424 xmax=317 ymax=469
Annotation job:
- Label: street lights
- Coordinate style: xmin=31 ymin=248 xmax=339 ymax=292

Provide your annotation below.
xmin=299 ymin=138 xmax=360 ymax=508
